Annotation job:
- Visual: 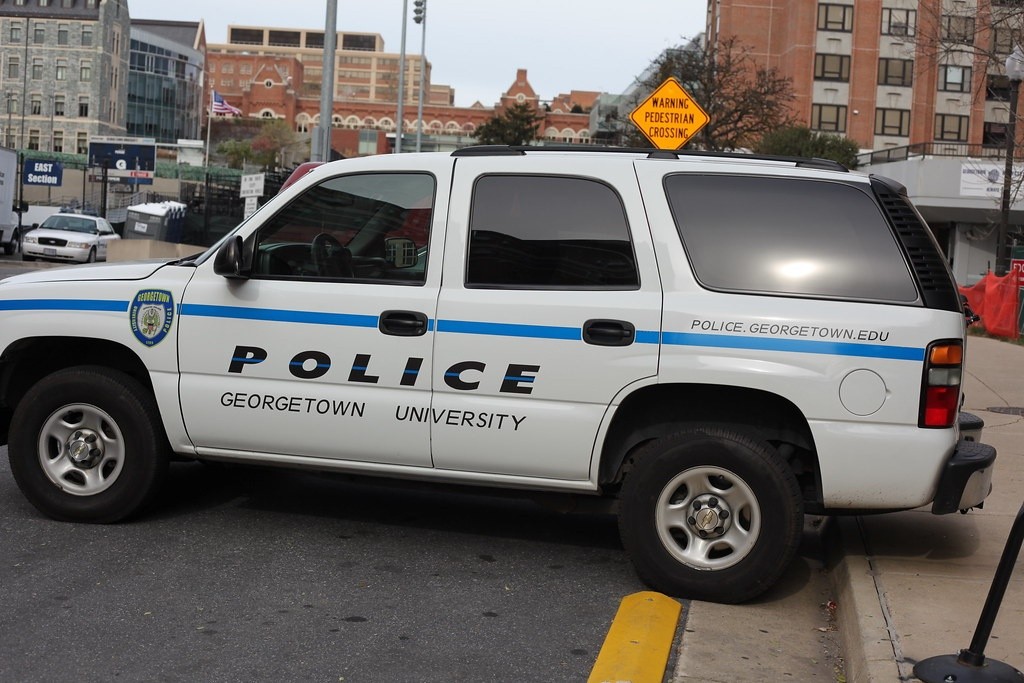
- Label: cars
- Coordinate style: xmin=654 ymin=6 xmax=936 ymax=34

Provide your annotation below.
xmin=267 ymin=160 xmax=434 ymax=253
xmin=21 ymin=212 xmax=121 ymax=264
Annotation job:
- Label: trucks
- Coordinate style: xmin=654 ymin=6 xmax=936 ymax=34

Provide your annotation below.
xmin=0 ymin=145 xmax=21 ymax=256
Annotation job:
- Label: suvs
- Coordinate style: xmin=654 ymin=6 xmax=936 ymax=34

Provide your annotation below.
xmin=0 ymin=143 xmax=999 ymax=604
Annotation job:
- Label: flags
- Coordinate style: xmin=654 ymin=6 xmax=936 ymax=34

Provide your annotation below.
xmin=212 ymin=91 xmax=243 ymax=115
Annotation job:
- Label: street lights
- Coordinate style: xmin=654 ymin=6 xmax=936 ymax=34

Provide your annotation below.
xmin=994 ymin=46 xmax=1023 ymax=279
